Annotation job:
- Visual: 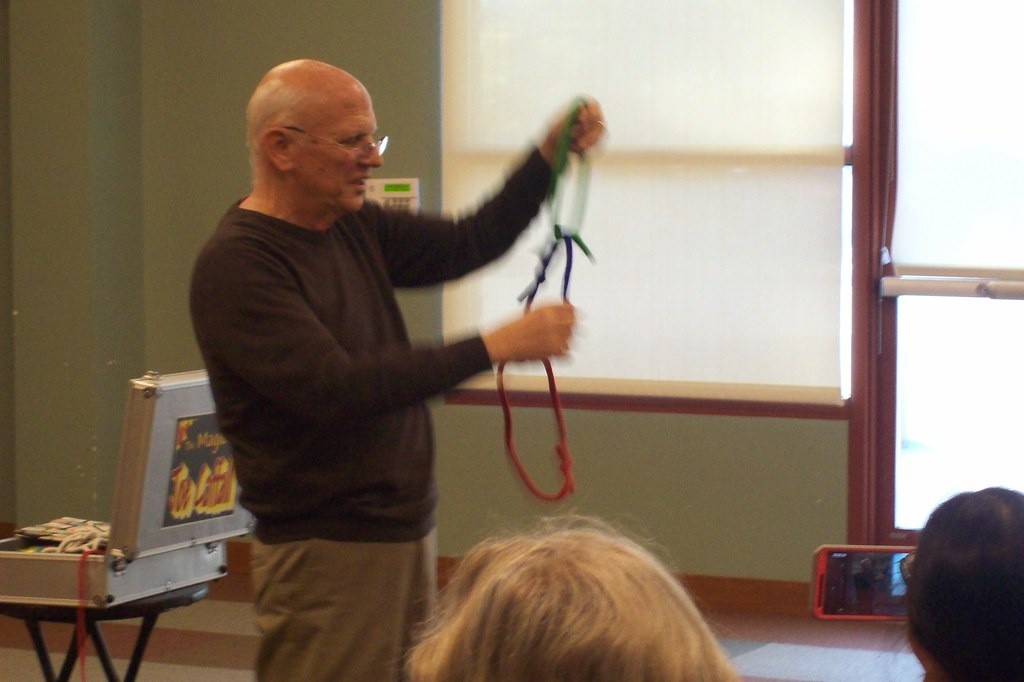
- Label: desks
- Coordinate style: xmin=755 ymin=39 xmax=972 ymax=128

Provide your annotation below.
xmin=0 ymin=582 xmax=207 ymax=682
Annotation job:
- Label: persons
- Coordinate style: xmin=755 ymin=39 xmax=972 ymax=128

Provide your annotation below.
xmin=189 ymin=59 xmax=606 ymax=682
xmin=908 ymin=488 xmax=1024 ymax=682
xmin=400 ymin=518 xmax=742 ymax=682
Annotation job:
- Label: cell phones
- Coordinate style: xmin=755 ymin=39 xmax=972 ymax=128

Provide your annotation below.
xmin=811 ymin=544 xmax=917 ymax=620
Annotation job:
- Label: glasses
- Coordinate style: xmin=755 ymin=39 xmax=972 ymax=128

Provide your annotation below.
xmin=899 ymin=549 xmax=920 ymax=595
xmin=280 ymin=125 xmax=389 ymax=162
